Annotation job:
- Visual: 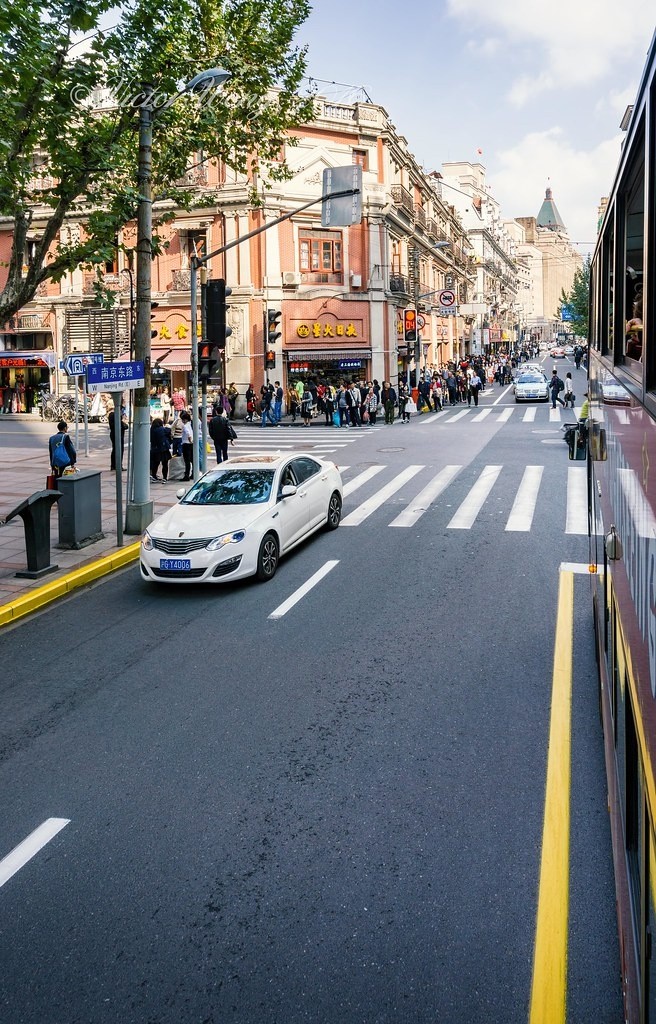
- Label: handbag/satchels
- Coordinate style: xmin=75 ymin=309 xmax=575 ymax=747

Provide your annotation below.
xmin=405 ymin=397 xmax=417 ymax=413
xmin=46 ymin=468 xmax=59 ymax=490
xmin=380 ymin=406 xmax=386 ymax=415
xmin=308 ymin=406 xmax=313 ymax=411
xmin=54 ymin=433 xmax=70 ymax=467
xmin=63 ymin=466 xmax=80 ymax=476
xmin=363 ymin=411 xmax=370 ymax=421
xmin=564 ymin=393 xmax=576 ymax=402
xmin=355 ymin=402 xmax=360 ymax=407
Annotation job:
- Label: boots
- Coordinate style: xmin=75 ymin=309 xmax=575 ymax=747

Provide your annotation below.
xmin=189 ymin=471 xmax=193 ymax=479
xmin=180 ymin=472 xmax=189 ymax=481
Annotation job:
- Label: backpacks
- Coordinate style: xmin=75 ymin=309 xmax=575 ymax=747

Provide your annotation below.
xmin=556 ymin=376 xmax=564 ymax=391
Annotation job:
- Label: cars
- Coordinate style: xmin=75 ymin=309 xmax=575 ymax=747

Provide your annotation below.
xmin=512 ymin=363 xmax=553 ymax=402
xmin=539 ymin=343 xmax=575 ymax=359
xmin=140 ymin=452 xmax=344 ymax=583
xmin=600 ymin=373 xmax=631 ymax=403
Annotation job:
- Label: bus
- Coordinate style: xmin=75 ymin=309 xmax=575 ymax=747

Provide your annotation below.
xmin=569 ymin=25 xmax=655 ymax=1024
xmin=558 ymin=333 xmax=585 ymax=346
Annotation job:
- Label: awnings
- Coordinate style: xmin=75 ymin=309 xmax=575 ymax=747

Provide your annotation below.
xmin=171 ymin=221 xmax=208 ymax=230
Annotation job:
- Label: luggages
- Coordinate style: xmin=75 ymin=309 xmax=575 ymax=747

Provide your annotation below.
xmin=332 ymin=402 xmax=341 ymax=428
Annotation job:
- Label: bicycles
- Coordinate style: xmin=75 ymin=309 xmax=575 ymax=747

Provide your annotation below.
xmin=42 ymin=393 xmax=75 ymax=423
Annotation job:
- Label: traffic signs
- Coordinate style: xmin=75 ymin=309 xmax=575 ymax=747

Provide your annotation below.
xmin=62 ymin=355 xmax=104 ymax=376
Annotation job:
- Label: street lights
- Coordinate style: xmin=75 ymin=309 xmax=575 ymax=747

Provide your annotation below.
xmin=456 ymin=274 xmax=479 ymax=368
xmin=415 ymin=241 xmax=451 ymax=387
xmin=124 ymin=66 xmax=235 ymax=534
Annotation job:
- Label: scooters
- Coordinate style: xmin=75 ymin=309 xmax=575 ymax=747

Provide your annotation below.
xmin=562 ymin=421 xmax=588 ymax=445
xmin=78 ymin=391 xmax=109 ymax=423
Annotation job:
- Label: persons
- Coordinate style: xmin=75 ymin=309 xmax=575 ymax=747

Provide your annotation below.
xmin=563 ymin=373 xmax=574 ymax=408
xmin=49 ymin=422 xmax=76 ymax=504
xmin=244 ymin=341 xmax=540 ymax=428
xmin=548 ymin=370 xmax=566 ymax=409
xmin=579 ymin=393 xmax=588 ymax=421
xmin=108 ymin=381 xmax=238 ymax=483
xmin=2 ymin=384 xmax=13 ymax=414
xmin=547 ymin=339 xmax=587 ymax=370
xmin=26 ymin=385 xmax=35 ymax=413
xmin=609 ymin=288 xmax=643 ymax=360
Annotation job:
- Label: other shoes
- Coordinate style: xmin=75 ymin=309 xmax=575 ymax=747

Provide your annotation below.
xmin=551 ymin=406 xmax=555 ymax=409
xmin=151 ymin=479 xmax=159 ymax=483
xmin=121 ymin=468 xmax=128 ymax=471
xmin=346 ymin=424 xmax=349 ymax=428
xmin=163 ymin=479 xmax=166 ymax=484
xmin=351 ymin=424 xmax=357 ymax=427
xmin=306 ymin=425 xmax=310 ymax=427
xmin=563 ymin=402 xmax=566 ymax=409
xmin=402 ymin=420 xmax=406 ymax=424
xmin=301 ymin=424 xmax=305 ymax=427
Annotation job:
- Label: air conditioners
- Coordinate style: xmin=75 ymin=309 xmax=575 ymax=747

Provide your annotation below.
xmin=282 ymin=271 xmax=300 ymax=286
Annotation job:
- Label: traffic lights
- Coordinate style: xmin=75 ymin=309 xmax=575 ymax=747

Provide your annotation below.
xmin=268 ymin=309 xmax=282 ymax=343
xmin=265 ymin=350 xmax=276 ymax=370
xmin=407 ymin=347 xmax=415 ymax=364
xmin=403 ymin=309 xmax=418 ymax=342
xmin=196 ymin=339 xmax=217 ymax=378
xmin=206 ymin=278 xmax=232 ymax=349
